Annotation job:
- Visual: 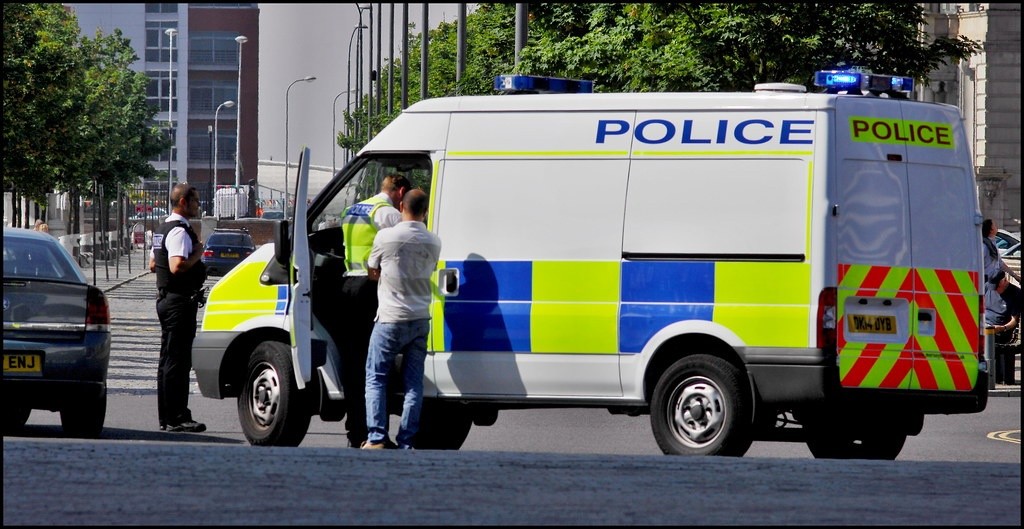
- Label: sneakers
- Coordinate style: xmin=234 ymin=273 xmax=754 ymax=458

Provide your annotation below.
xmin=160 ymin=419 xmax=207 ymax=433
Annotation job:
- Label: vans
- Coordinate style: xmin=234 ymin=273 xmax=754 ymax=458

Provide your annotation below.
xmin=190 ymin=65 xmax=995 ymax=461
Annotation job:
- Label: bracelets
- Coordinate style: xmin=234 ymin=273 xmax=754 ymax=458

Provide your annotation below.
xmin=1003 ymin=325 xmax=1007 ymax=333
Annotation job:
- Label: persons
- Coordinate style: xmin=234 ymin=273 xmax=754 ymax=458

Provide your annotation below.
xmin=360 ymin=189 xmax=443 ymax=450
xmin=147 ymin=183 xmax=207 ymax=433
xmin=339 ymin=173 xmax=412 ymax=448
xmin=981 ymin=219 xmax=1024 ymax=386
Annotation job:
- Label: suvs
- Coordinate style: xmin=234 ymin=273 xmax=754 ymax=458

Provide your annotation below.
xmin=200 ymin=227 xmax=257 ymax=278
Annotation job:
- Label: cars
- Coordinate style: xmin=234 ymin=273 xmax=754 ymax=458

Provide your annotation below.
xmin=989 ymin=228 xmax=1022 ymax=279
xmin=4 ymin=227 xmax=112 ymax=438
xmin=130 ymin=207 xmax=166 ymax=220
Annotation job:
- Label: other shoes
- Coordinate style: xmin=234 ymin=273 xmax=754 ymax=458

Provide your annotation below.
xmin=360 ymin=441 xmax=387 ymax=449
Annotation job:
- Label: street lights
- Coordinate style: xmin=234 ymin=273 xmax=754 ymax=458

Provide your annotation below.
xmin=333 ymin=88 xmax=359 ymax=177
xmin=235 ymin=35 xmax=249 ymax=217
xmin=213 ymin=101 xmax=235 ymax=216
xmin=345 ymin=21 xmax=369 ymax=162
xmin=283 ymin=75 xmax=317 ymax=219
xmin=165 ymin=27 xmax=179 ymax=216
xmin=355 ymin=4 xmax=372 ymax=153
